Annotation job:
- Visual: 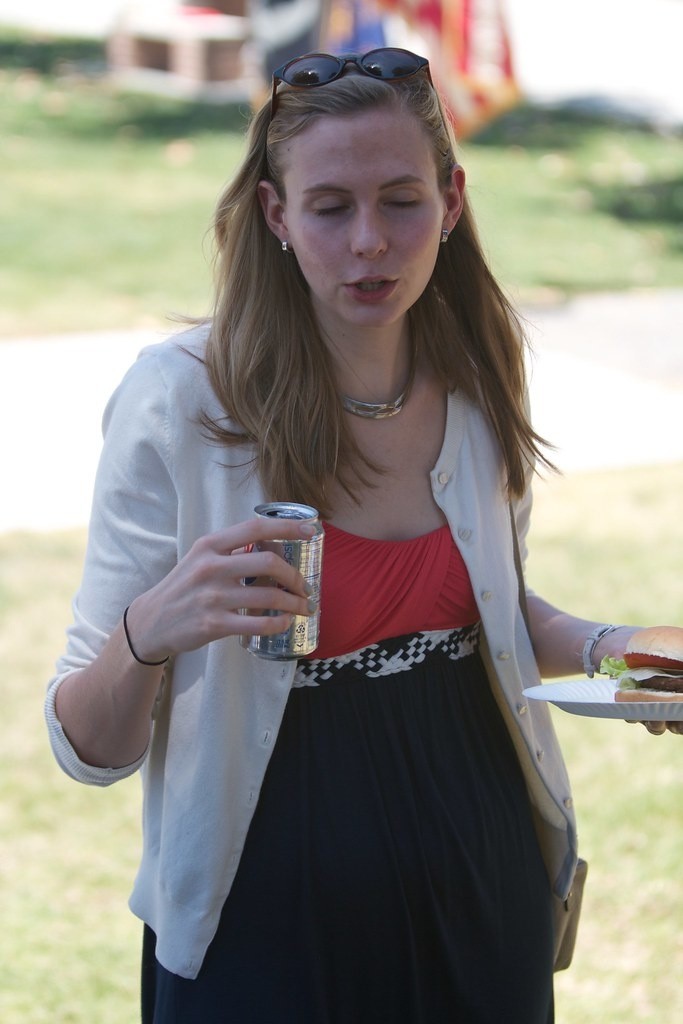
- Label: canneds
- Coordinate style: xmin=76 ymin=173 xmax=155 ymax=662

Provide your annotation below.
xmin=237 ymin=501 xmax=325 ymax=660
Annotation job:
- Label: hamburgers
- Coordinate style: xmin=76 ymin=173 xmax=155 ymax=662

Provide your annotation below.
xmin=597 ymin=626 xmax=683 ymax=703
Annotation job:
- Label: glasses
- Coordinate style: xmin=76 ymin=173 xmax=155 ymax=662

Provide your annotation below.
xmin=273 ymin=47 xmax=433 ymax=117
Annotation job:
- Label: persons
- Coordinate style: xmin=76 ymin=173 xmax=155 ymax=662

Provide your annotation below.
xmin=45 ymin=55 xmax=683 ymax=1024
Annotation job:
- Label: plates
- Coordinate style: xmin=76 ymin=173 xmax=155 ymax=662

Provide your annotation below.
xmin=522 ymin=679 xmax=683 ymax=721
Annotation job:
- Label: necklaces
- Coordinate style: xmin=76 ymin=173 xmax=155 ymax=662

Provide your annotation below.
xmin=330 ymin=309 xmax=418 ymax=420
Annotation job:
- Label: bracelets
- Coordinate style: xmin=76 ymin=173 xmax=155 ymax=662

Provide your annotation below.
xmin=583 ymin=624 xmax=626 ymax=675
xmin=118 ymin=605 xmax=170 ymax=666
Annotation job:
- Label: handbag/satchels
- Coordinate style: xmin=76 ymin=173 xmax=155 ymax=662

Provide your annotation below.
xmin=548 ymin=857 xmax=589 ymax=975
